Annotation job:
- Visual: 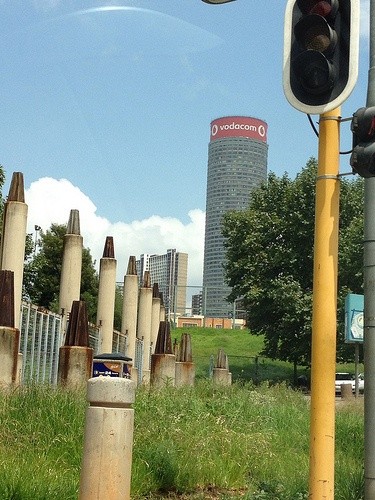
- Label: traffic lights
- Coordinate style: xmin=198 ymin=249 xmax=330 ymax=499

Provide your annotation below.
xmin=351 ymin=107 xmax=375 ymax=177
xmin=282 ymin=0 xmax=361 ymax=113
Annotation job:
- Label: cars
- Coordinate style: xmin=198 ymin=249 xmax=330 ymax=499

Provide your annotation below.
xmin=354 ymin=372 xmax=365 ymax=392
xmin=334 ymin=372 xmax=356 ymax=394
